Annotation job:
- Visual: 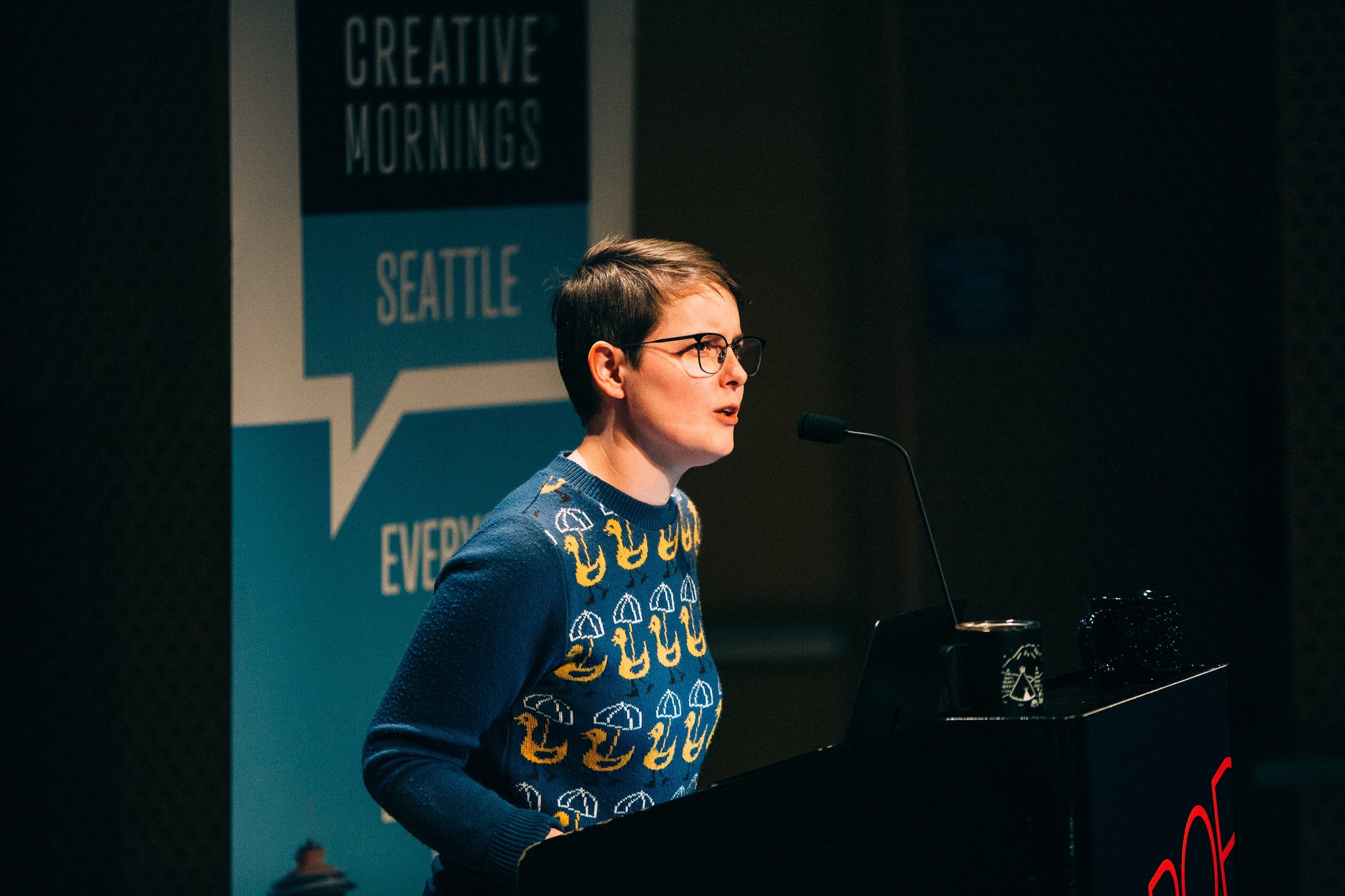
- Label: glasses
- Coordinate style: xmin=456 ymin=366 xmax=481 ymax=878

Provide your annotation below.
xmin=611 ymin=333 xmax=766 ymax=376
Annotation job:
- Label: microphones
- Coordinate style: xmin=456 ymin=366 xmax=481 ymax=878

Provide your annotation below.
xmin=796 ymin=414 xmax=958 ymax=630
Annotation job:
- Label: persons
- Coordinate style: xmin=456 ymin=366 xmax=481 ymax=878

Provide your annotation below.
xmin=361 ymin=231 xmax=764 ymax=896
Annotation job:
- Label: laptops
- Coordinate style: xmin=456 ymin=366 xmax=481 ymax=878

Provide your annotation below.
xmin=707 ymin=596 xmax=968 ymax=789
